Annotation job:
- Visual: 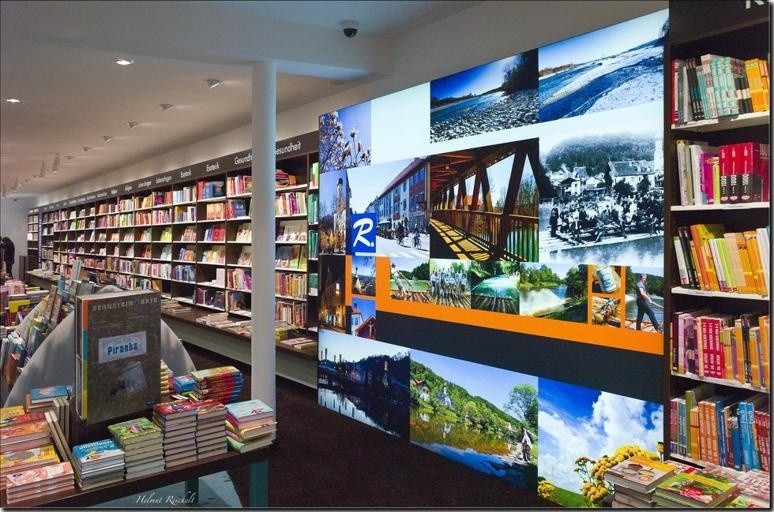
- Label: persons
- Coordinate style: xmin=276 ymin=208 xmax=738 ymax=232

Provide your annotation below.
xmin=550 ymin=189 xmax=664 ymax=242
xmin=391 ymin=264 xmax=411 ymax=300
xmin=636 ymin=273 xmax=661 ymax=332
xmin=431 ymin=267 xmax=468 ymax=299
xmin=395 ymin=222 xmax=421 ymax=248
xmin=0 ymin=237 xmax=17 ymax=281
xmin=518 ymin=426 xmax=537 ymax=462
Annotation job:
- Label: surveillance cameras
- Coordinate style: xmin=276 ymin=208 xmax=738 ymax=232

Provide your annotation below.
xmin=340 ymin=20 xmax=360 ymax=40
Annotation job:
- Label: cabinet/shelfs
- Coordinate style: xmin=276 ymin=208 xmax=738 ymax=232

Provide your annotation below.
xmin=662 ymin=1 xmax=770 ymax=484
xmin=1 ymin=446 xmax=272 ymax=510
xmin=19 ymin=128 xmax=320 ymax=394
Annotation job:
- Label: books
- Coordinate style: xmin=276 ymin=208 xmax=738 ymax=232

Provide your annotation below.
xmin=108 ymin=203 xmax=118 ymax=212
xmin=161 ymin=358 xmax=175 ymax=396
xmin=152 ymin=280 xmax=170 ymax=293
xmin=190 ymin=366 xmax=244 ymax=403
xmin=670 ymin=383 xmax=770 ymax=472
xmin=54 ymin=210 xmax=68 ymax=230
xmin=110 ymin=231 xmax=119 ymax=241
xmin=107 ymin=214 xmax=119 ymax=226
xmin=29 ymin=225 xmax=33 ymax=231
xmin=276 ymin=299 xmax=307 ymax=327
xmin=89 ymin=231 xmax=95 ymax=240
xmin=98 ymin=231 xmax=106 ymax=241
xmin=135 ymin=211 xmax=151 ymax=224
xmin=275 ymin=191 xmax=307 ymax=216
xmin=308 ymin=229 xmax=318 ymax=260
xmin=71 ymin=289 xmax=162 ymax=425
xmin=49 ymin=213 xmax=54 ymax=222
xmin=677 ymin=140 xmax=768 ymax=204
xmin=107 ymin=258 xmax=119 ymax=271
xmin=159 ymin=226 xmax=172 ymax=241
xmin=41 ymin=248 xmax=60 ymax=271
xmin=0 ymin=384 xmax=75 ymax=508
xmin=89 ymin=207 xmax=95 ymax=215
xmin=227 ymin=268 xmax=251 ymax=290
xmin=113 ymin=244 xmax=119 ymax=255
xmin=34 ymin=233 xmax=38 ymax=240
xmin=204 ymin=224 xmax=225 ymax=241
xmin=205 ymin=203 xmax=225 ymax=220
xmin=276 ymin=169 xmax=296 ymax=187
xmin=97 ymin=203 xmax=106 ymax=213
xmin=139 ymin=227 xmax=151 ymax=241
xmin=275 ymin=244 xmax=307 ymax=271
xmin=69 ymin=221 xmax=76 ymax=229
xmin=277 ymin=220 xmax=307 ymax=240
xmin=43 ymin=214 xmax=48 ymax=222
xmin=197 ymin=287 xmax=225 ymax=309
xmin=225 ymin=291 xmax=247 ymax=312
xmin=275 ymin=272 xmax=307 ymax=298
xmin=119 ymin=196 xmax=134 ymax=211
xmin=119 ymin=259 xmax=151 ymax=275
xmin=27 ymin=269 xmax=60 ymax=281
xmin=109 ymin=418 xmax=165 ymax=481
xmin=97 ymin=216 xmax=106 ymax=227
xmin=34 ymin=216 xmax=38 ymax=222
xmin=99 ymin=272 xmax=151 ymax=290
xmin=226 ymin=175 xmax=252 ymax=196
xmin=672 ymin=223 xmax=770 ymax=296
xmin=49 ymin=226 xmax=53 ymax=234
xmin=76 ymin=234 xmax=84 ymax=241
xmin=119 ymin=213 xmax=134 ymax=226
xmin=99 ymin=247 xmax=106 ymax=254
xmin=79 ymin=246 xmax=84 ymax=253
xmin=140 ymin=244 xmax=151 ymax=258
xmin=197 ymin=180 xmax=224 ymax=200
xmin=160 ymin=245 xmax=171 ymax=260
xmin=69 ymin=210 xmax=76 ymax=218
xmin=174 ymin=206 xmax=196 ymax=222
xmin=125 ymin=244 xmax=134 ymax=256
xmin=161 ymin=297 xmax=191 ymax=314
xmin=152 ymin=400 xmax=197 ymax=469
xmin=90 ymin=247 xmax=94 ymax=253
xmin=172 ymin=185 xmax=196 ymax=204
xmin=174 ymin=374 xmax=197 ymax=395
xmin=34 ymin=225 xmax=38 ymax=231
xmin=0 ymin=286 xmax=74 ymax=387
xmin=202 ymin=246 xmax=225 ymax=263
xmin=225 ymin=399 xmax=277 ymax=454
xmin=77 ymin=220 xmax=85 ymax=229
xmin=309 ymin=273 xmax=319 ymax=296
xmin=27 ymin=233 xmax=33 ymax=240
xmin=152 ymin=191 xmax=172 ymax=206
xmin=670 ymin=308 xmax=770 ymax=390
xmin=212 ymin=269 xmax=225 ymax=287
xmin=236 ymin=223 xmax=252 ymax=241
xmin=78 ymin=209 xmax=85 ymax=217
xmin=123 ymin=228 xmax=134 ymax=241
xmin=227 ymin=197 xmax=253 ymax=219
xmin=135 ymin=193 xmax=152 ymax=209
xmin=307 ymin=194 xmax=318 ymax=225
xmin=237 ymin=246 xmax=252 ymax=265
xmin=276 ymin=327 xmax=318 ymax=349
xmin=172 ymin=265 xmax=196 ymax=282
xmin=60 ymin=254 xmax=105 ymax=295
xmin=181 ymin=225 xmax=196 ymax=241
xmin=196 ymin=312 xmax=251 ymax=338
xmin=191 ymin=397 xmax=228 ymax=461
xmin=0 ymin=270 xmax=28 ymax=288
xmin=604 ymin=455 xmax=772 ymax=509
xmin=28 ymin=216 xmax=33 ymax=223
xmin=672 ymin=54 xmax=769 ymax=123
xmin=43 ymin=227 xmax=47 ymax=234
xmin=179 ymin=245 xmax=196 ymax=260
xmin=309 ymin=162 xmax=319 ymax=189
xmin=71 ymin=438 xmax=125 ymax=492
xmin=152 ymin=263 xmax=171 ymax=279
xmin=88 ymin=220 xmax=95 ymax=227
xmin=152 ymin=208 xmax=173 ymax=224
